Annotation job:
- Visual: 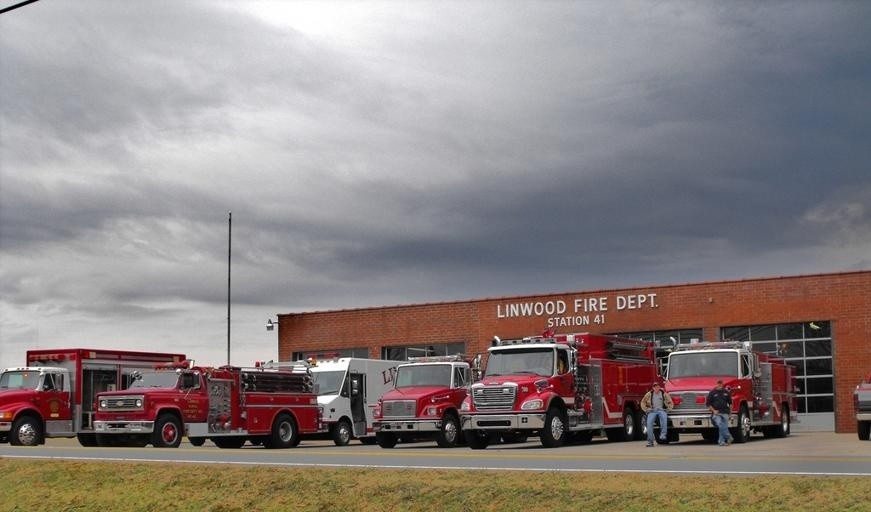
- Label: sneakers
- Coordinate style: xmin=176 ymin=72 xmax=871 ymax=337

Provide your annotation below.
xmin=719 ymin=441 xmax=726 ymax=446
xmin=645 ymin=443 xmax=654 ymax=447
xmin=727 ymin=437 xmax=734 ymax=445
xmin=659 ymin=436 xmax=666 ymax=439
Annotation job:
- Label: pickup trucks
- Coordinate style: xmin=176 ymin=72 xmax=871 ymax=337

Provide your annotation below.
xmin=853 ymin=380 xmax=871 ymax=440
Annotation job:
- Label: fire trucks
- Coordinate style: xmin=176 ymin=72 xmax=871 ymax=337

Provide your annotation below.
xmin=372 ymin=353 xmax=485 ymax=448
xmin=93 ymin=360 xmax=322 ymax=448
xmin=653 ymin=336 xmax=797 ymax=445
xmin=0 ymin=349 xmax=186 ymax=446
xmin=457 ymin=332 xmax=659 ymax=448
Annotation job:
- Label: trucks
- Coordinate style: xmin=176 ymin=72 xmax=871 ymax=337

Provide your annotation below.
xmin=291 ymin=358 xmax=410 ymax=446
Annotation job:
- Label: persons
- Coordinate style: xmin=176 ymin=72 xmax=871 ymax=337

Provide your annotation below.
xmin=545 ymin=352 xmax=564 ymax=374
xmin=706 ymin=379 xmax=734 ymax=446
xmin=640 ymin=382 xmax=674 ymax=447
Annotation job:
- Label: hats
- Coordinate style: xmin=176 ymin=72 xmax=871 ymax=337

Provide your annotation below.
xmin=653 ymin=382 xmax=660 ymax=386
